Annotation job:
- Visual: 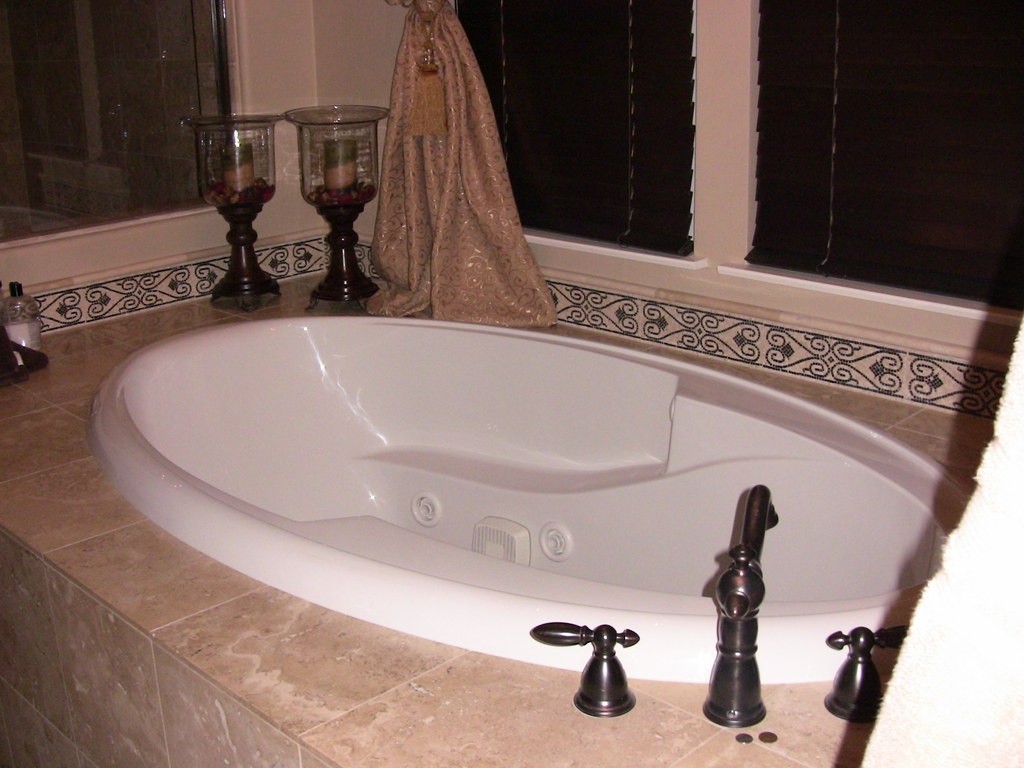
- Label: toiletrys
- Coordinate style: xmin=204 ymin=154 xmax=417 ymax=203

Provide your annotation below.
xmin=0 ymin=282 xmax=44 ymax=366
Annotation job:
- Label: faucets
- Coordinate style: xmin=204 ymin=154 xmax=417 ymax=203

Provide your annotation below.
xmin=700 ymin=483 xmax=779 ymax=728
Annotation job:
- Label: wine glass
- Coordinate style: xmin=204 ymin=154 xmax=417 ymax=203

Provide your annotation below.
xmin=180 ymin=105 xmax=390 ymax=306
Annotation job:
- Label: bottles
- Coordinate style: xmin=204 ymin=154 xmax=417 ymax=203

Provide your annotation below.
xmin=1 ymin=282 xmax=42 ymax=352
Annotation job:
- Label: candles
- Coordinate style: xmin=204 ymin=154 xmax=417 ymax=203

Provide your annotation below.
xmin=324 ymin=139 xmax=357 ymax=190
xmin=222 ymin=141 xmax=254 ymax=192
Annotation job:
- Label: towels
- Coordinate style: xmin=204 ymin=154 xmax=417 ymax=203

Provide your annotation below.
xmin=859 ymin=304 xmax=1023 ymax=768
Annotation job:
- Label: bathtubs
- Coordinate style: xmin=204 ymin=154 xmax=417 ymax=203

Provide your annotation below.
xmin=93 ymin=308 xmax=975 ymax=685
